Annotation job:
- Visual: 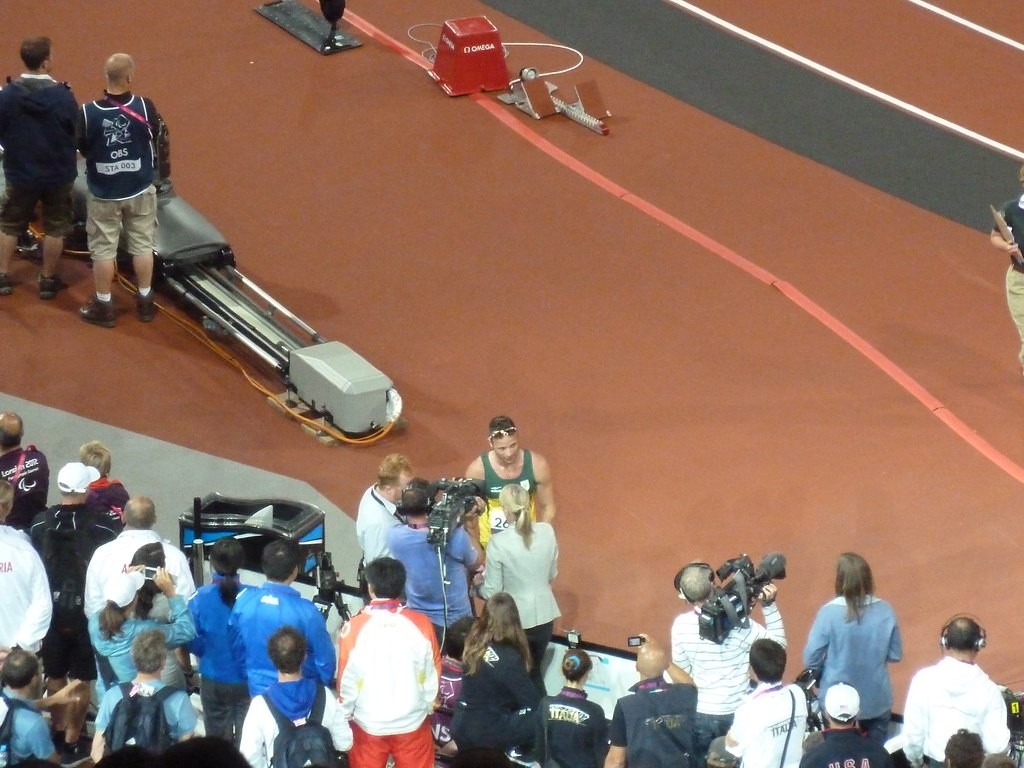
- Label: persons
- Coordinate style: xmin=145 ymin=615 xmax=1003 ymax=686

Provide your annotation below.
xmin=77 ymin=52 xmax=158 ymax=326
xmin=0 ymin=37 xmax=83 ymax=301
xmin=228 ymin=414 xmax=609 ymax=768
xmin=605 ymin=550 xmax=1024 ymax=768
xmin=990 ymin=164 xmax=1024 ymax=370
xmin=0 ymin=411 xmax=254 ymax=768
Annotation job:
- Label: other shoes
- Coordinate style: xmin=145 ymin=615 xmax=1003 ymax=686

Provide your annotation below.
xmin=504 ymin=744 xmax=536 ymax=767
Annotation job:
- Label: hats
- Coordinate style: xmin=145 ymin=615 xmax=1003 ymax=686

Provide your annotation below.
xmin=825 ymin=682 xmax=860 ymax=722
xmin=58 ymin=463 xmax=100 ymax=493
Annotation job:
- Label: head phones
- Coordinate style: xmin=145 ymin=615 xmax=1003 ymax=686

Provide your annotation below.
xmin=396 ymin=484 xmax=437 ymax=515
xmin=940 ymin=612 xmax=986 ymax=652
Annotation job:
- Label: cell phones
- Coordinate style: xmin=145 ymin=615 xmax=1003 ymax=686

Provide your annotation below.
xmin=143 ymin=567 xmax=158 ymax=580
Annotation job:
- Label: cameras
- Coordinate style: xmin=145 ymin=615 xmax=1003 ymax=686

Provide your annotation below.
xmin=628 ymin=636 xmax=646 ymax=647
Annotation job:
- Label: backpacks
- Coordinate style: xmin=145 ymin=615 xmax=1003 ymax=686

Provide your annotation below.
xmin=0 ymin=695 xmax=39 ymax=768
xmin=104 ymin=681 xmax=181 ymax=754
xmin=262 ymin=683 xmax=340 ymax=768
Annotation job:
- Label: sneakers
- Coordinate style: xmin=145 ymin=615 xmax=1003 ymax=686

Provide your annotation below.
xmin=52 ymin=731 xmax=67 ymax=754
xmin=78 ymin=295 xmax=116 ymax=326
xmin=1 ymin=275 xmax=13 ymax=294
xmin=38 ymin=276 xmax=62 ymax=298
xmin=136 ymin=288 xmax=156 ymax=322
xmin=58 ymin=740 xmax=93 ymax=768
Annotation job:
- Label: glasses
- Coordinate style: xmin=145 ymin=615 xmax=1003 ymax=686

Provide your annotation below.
xmin=488 ymin=429 xmax=516 ymax=439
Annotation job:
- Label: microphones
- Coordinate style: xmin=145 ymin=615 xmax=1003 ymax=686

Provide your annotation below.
xmin=754 ymin=552 xmax=786 ymax=581
